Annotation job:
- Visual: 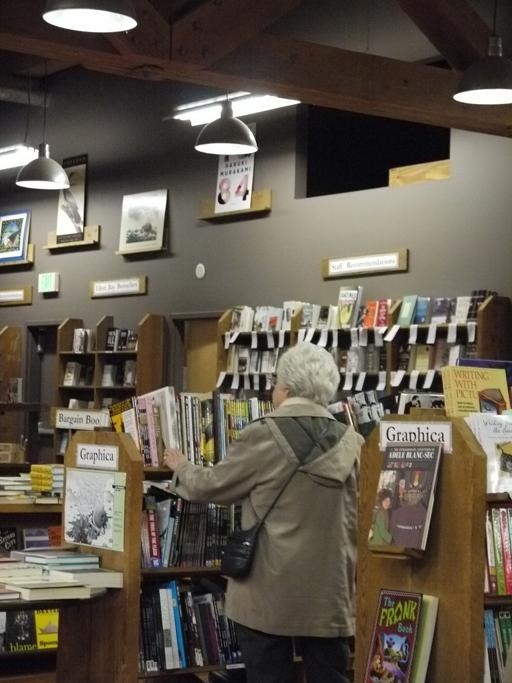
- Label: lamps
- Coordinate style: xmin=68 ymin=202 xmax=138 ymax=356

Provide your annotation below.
xmin=14 ymin=58 xmax=72 ymax=190
xmin=450 ymin=1 xmax=512 ymax=107
xmin=0 ymin=70 xmax=42 ymax=172
xmin=193 ymin=87 xmax=261 ymax=159
xmin=159 ymin=85 xmax=300 ymax=128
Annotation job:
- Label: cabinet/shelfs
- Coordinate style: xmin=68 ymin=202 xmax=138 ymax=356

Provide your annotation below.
xmin=0 ymin=290 xmax=512 ymax=683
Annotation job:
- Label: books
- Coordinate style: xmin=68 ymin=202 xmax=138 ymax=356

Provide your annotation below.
xmin=1 ymin=464 xmax=64 ymax=505
xmin=64 ymin=328 xmax=139 ymax=408
xmin=2 ymin=526 xmax=124 ymax=600
xmin=109 ymin=386 xmax=242 ymax=681
xmin=363 ymin=585 xmax=438 ymax=683
xmin=6 ymin=377 xmax=24 ymax=401
xmin=222 ymin=285 xmax=497 ymax=427
xmin=438 ymin=353 xmax=510 ymax=682
xmin=365 ymin=440 xmax=443 ymax=551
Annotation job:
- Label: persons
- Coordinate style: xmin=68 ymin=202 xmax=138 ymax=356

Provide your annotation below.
xmin=159 ymin=343 xmax=369 ymax=682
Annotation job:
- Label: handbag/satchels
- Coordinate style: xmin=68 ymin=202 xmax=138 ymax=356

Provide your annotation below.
xmin=218 ymin=527 xmax=260 ymax=578
